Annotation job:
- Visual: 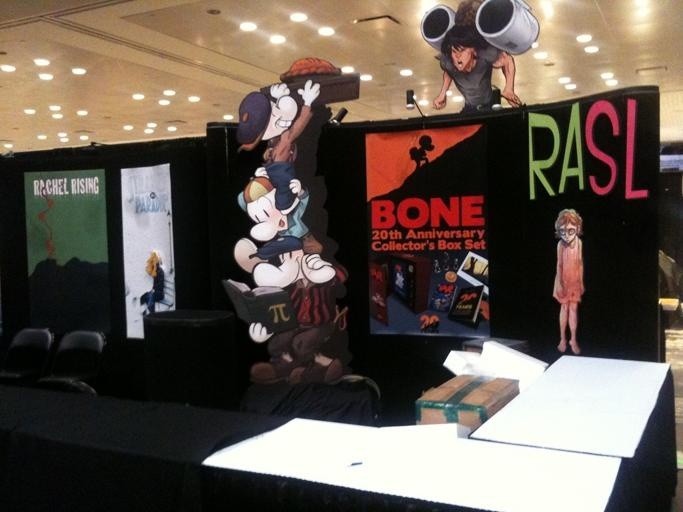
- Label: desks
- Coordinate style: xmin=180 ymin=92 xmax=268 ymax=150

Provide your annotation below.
xmin=602 ymin=368 xmax=677 ymax=510
xmin=1 ymin=385 xmax=474 ymax=510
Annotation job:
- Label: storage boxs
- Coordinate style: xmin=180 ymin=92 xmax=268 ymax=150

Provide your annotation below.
xmin=416 ymin=371 xmax=519 ymax=432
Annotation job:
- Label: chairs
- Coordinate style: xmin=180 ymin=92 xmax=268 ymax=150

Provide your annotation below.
xmin=0 ymin=327 xmax=108 ymax=396
